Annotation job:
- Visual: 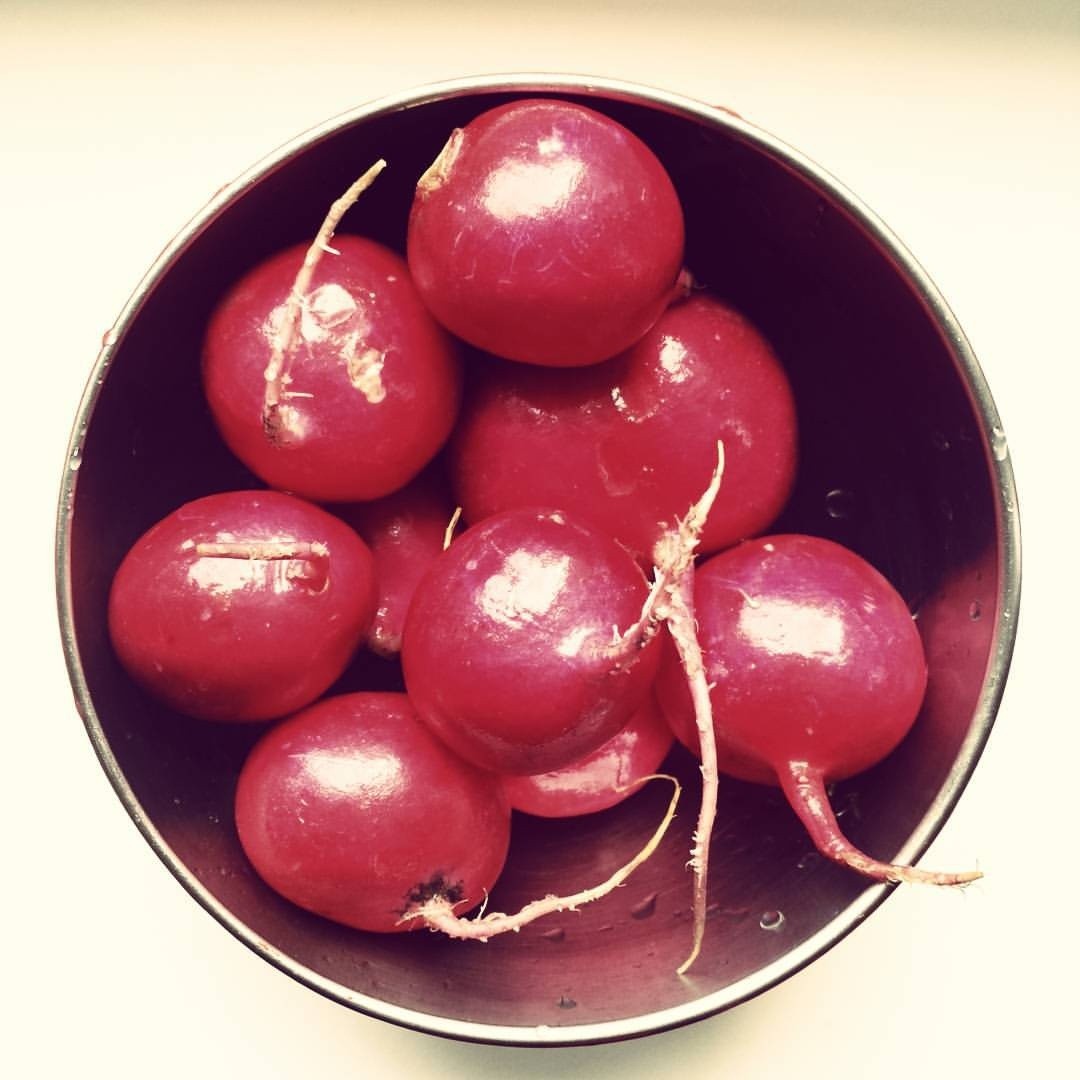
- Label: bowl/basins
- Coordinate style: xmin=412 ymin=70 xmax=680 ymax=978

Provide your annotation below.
xmin=50 ymin=70 xmax=1023 ymax=1050
